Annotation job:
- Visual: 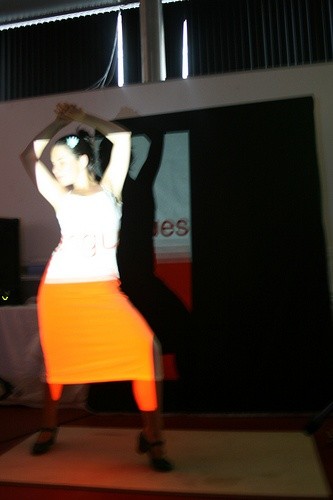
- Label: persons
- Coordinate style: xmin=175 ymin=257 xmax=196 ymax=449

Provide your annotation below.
xmin=19 ymin=103 xmax=175 ymax=472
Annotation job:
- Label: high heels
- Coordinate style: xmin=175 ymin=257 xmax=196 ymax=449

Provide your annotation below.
xmin=31 ymin=422 xmax=61 ymax=454
xmin=135 ymin=433 xmax=172 ymax=472
